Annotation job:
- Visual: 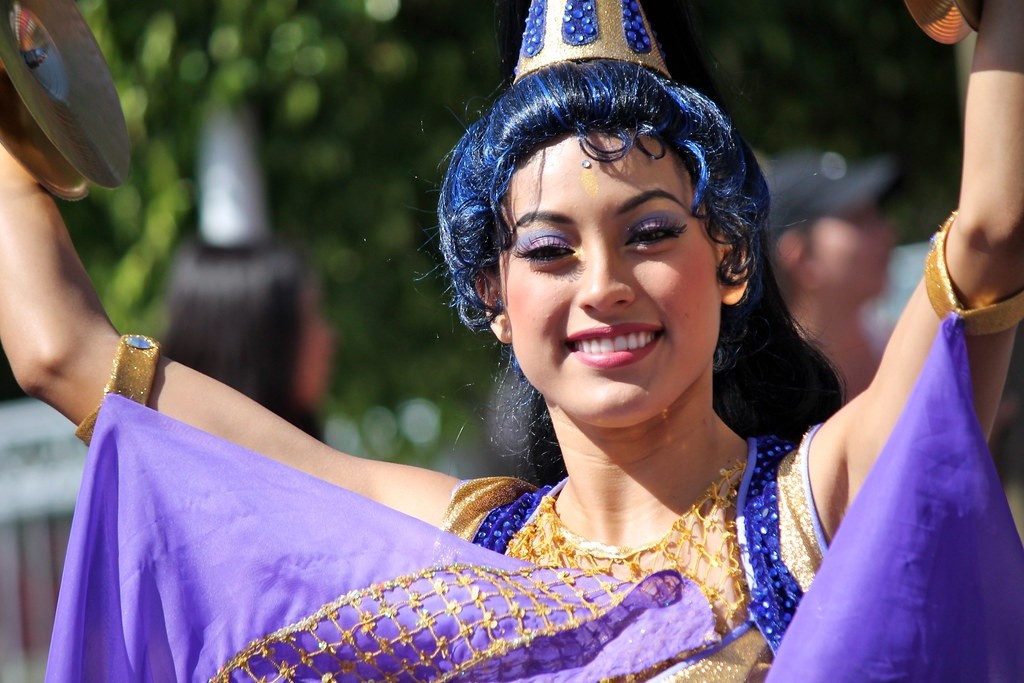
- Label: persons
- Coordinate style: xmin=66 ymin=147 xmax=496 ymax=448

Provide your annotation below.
xmin=161 ymin=264 xmax=325 ymax=448
xmin=0 ymin=0 xmax=1024 ymax=683
xmin=759 ymin=160 xmax=933 ymax=404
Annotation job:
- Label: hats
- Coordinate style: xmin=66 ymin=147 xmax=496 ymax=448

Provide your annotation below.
xmin=766 ymin=154 xmax=896 ymax=232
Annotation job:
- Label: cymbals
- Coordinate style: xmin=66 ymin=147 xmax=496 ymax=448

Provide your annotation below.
xmin=905 ymin=0 xmax=987 ymax=46
xmin=0 ymin=0 xmax=132 ymax=201
